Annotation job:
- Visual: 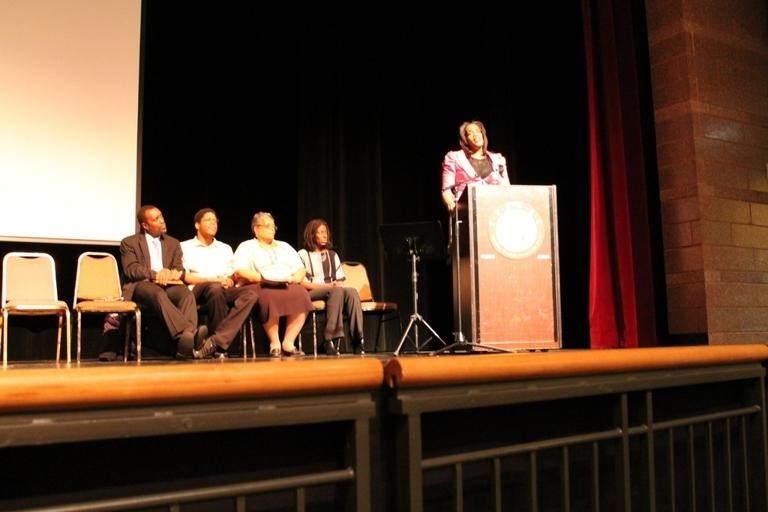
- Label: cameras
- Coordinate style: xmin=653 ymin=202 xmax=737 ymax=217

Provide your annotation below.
xmin=398 ymin=236 xmax=423 ymax=255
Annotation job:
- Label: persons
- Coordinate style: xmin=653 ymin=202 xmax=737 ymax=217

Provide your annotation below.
xmin=234 ymin=211 xmax=313 ymax=357
xmin=97 ymin=313 xmax=132 ymax=361
xmin=440 ymin=120 xmax=511 ymax=213
xmin=180 ymin=208 xmax=259 ymax=358
xmin=119 ymin=205 xmax=209 ymax=359
xmin=298 ymin=219 xmax=365 ymax=356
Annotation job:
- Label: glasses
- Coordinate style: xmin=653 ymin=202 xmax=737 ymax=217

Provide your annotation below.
xmin=255 ymin=223 xmax=278 ymax=231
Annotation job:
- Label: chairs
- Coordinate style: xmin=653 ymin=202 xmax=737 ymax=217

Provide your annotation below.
xmin=341 ymin=261 xmax=402 ymax=354
xmin=196 ymin=305 xmax=247 ymax=358
xmin=0 ymin=252 xmax=72 ymax=368
xmin=250 ymin=306 xmax=304 ymax=359
xmin=124 ymin=306 xmax=171 ymax=363
xmin=310 ymin=301 xmax=326 ymax=358
xmin=73 ymin=252 xmax=144 ymax=365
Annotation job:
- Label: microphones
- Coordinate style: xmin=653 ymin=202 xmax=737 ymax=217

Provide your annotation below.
xmin=479 ymin=165 xmax=491 ymax=179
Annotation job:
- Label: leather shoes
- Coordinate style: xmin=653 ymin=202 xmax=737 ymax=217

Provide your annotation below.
xmin=175 ymin=327 xmax=195 ymax=360
xmin=194 ymin=324 xmax=209 ymax=351
xmin=269 ymin=347 xmax=282 ymax=357
xmin=354 ymin=343 xmax=364 ymax=355
xmin=326 ymin=341 xmax=339 ymax=356
xmin=282 ymin=345 xmax=306 ymax=357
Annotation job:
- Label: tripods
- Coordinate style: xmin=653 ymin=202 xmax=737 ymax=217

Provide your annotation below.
xmin=430 ymin=186 xmax=517 ymax=356
xmin=393 ymin=255 xmax=451 ymax=355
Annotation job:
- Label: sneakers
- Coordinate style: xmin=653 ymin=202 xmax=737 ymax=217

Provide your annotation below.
xmin=192 ymin=337 xmax=217 ymax=359
xmin=214 ymin=348 xmax=230 ymax=358
